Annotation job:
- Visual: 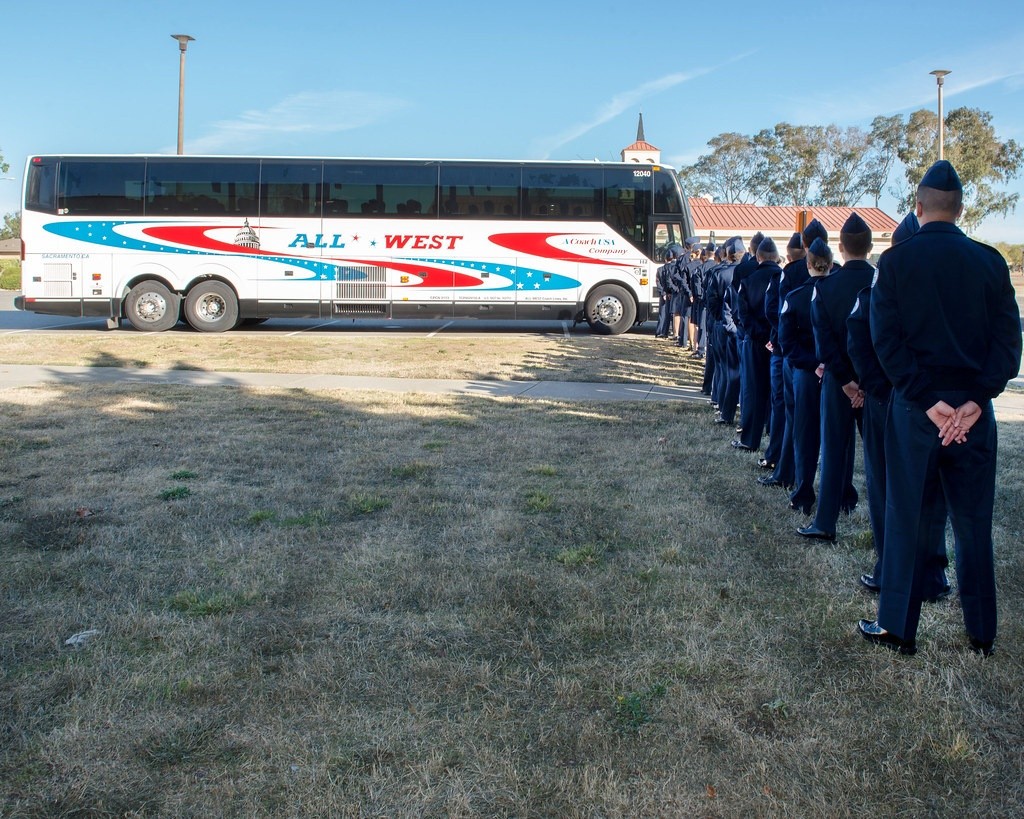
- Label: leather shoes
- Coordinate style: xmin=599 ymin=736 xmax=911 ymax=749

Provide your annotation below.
xmin=668 ymin=332 xmax=777 ymax=470
xmin=788 ymin=501 xmax=808 ymax=517
xmin=758 ymin=473 xmax=793 ymax=489
xmin=970 ymin=639 xmax=995 ymax=656
xmin=936 ymin=583 xmax=951 ymax=600
xmin=797 ymin=523 xmax=838 ymax=545
xmin=861 ymin=574 xmax=881 ymax=594
xmin=857 ymin=618 xmax=917 ymax=656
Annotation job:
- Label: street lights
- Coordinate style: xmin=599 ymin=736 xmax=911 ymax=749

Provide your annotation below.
xmin=930 ymin=69 xmax=952 ymax=162
xmin=170 ymin=33 xmax=197 ymax=199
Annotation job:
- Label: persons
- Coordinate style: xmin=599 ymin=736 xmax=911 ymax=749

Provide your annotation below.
xmin=848 ymin=159 xmax=1022 ymax=653
xmin=699 ymin=235 xmax=752 ymax=423
xmin=729 ymin=231 xmax=799 ymax=450
xmin=758 ymin=211 xmax=875 ymax=541
xmin=654 ymin=236 xmax=716 ymax=359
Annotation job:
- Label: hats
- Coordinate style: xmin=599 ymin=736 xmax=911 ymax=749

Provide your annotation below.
xmin=843 ymin=213 xmax=868 ymax=233
xmin=810 ymin=236 xmax=829 ymax=256
xmin=893 ymin=212 xmax=921 ymax=243
xmin=804 ymin=219 xmax=826 ymax=238
xmin=788 ymin=232 xmax=805 ymax=249
xmin=919 ymin=159 xmax=963 ymax=192
xmin=662 ymin=233 xmax=776 ymax=257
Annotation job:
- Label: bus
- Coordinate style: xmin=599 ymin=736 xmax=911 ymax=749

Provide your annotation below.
xmin=13 ymin=153 xmax=695 ymax=334
